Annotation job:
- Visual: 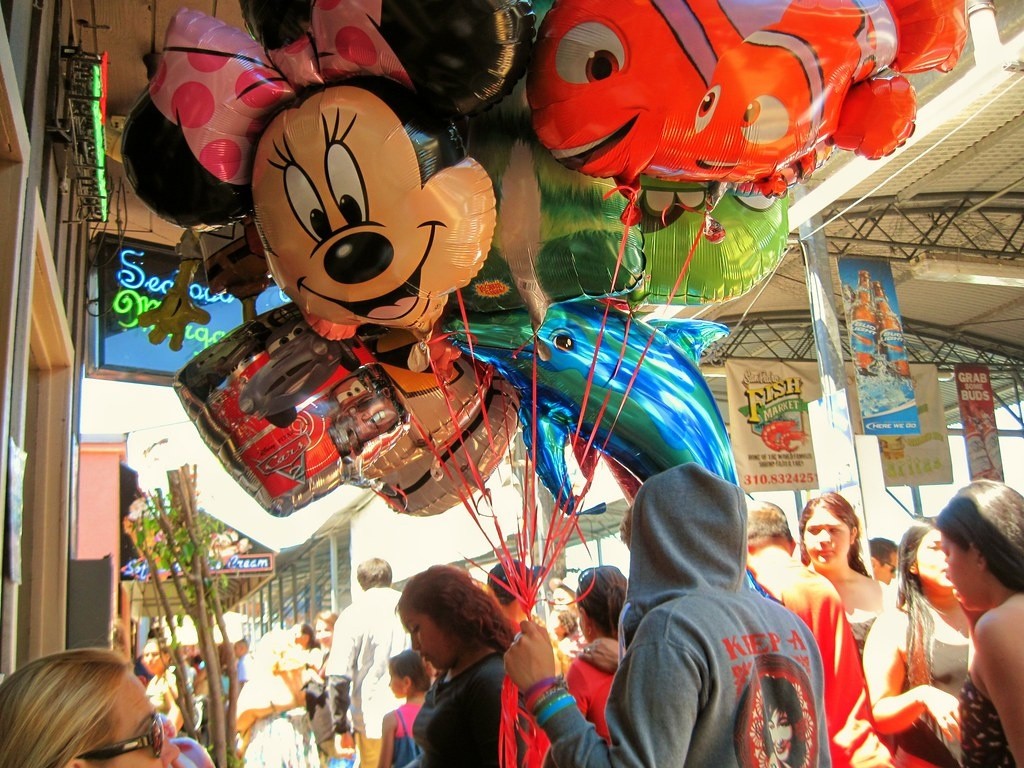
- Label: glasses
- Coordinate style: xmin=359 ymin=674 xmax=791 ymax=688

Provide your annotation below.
xmin=76 ymin=712 xmax=163 ymax=762
xmin=578 ymin=567 xmax=592 ymax=608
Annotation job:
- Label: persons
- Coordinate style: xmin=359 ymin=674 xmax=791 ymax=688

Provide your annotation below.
xmin=869 ymin=537 xmax=900 ymax=585
xmin=549 ymin=565 xmax=628 ymax=751
xmin=135 ymin=626 xmax=185 ymax=738
xmin=746 ymin=500 xmax=897 ymax=768
xmin=798 ymin=492 xmax=894 ymax=665
xmin=490 ymin=560 xmax=547 ymax=623
xmin=863 ymin=515 xmax=969 ymax=768
xmin=395 ymin=564 xmax=531 ymax=768
xmin=937 ymin=480 xmax=1024 ymax=768
xmin=0 ymin=648 xmax=184 ymax=768
xmin=186 ymin=558 xmax=432 ymax=768
xmin=504 ymin=462 xmax=832 ymax=768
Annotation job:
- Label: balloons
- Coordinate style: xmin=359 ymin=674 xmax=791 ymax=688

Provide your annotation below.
xmin=122 ymin=0 xmax=971 ymax=516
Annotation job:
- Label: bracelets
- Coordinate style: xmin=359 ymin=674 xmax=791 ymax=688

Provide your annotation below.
xmin=525 ymin=675 xmax=575 ymax=726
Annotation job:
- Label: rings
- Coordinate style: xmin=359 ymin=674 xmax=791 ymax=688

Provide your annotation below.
xmin=586 ymin=648 xmax=591 ymax=653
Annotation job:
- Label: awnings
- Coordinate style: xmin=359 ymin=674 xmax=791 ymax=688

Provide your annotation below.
xmin=122 ymin=487 xmax=277 ymax=626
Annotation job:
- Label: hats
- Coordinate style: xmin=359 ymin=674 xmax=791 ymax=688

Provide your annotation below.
xmin=487 ymin=561 xmax=546 ymax=602
